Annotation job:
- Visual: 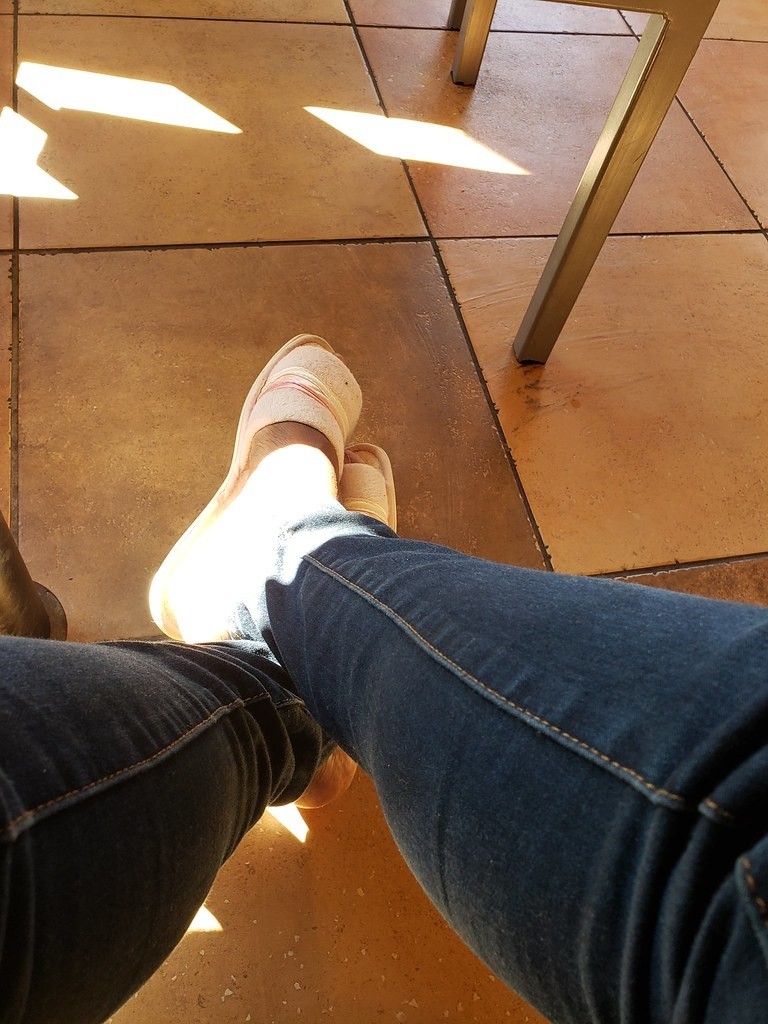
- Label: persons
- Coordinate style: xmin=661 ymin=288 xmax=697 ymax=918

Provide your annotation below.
xmin=0 ymin=333 xmax=768 ymax=1024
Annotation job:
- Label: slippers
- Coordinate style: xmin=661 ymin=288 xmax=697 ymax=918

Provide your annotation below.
xmin=150 ymin=333 xmax=395 ymax=809
